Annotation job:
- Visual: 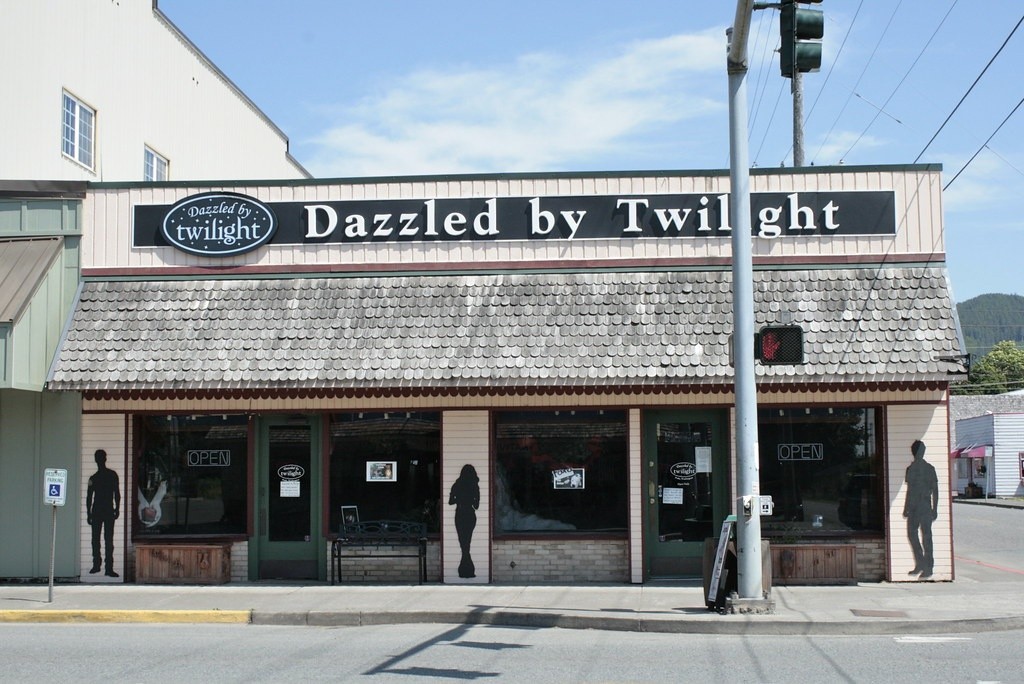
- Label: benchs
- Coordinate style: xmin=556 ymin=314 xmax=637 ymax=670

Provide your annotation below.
xmin=330 ymin=518 xmax=429 ymax=585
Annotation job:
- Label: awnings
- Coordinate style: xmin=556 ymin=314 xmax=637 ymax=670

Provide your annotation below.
xmin=961 ymin=444 xmax=993 ymax=457
xmin=950 ymin=447 xmax=972 ymax=460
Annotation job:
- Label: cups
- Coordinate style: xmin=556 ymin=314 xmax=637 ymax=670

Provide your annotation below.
xmin=812 ymin=515 xmax=823 ymax=527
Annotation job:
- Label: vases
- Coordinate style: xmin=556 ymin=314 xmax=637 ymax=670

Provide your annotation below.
xmin=134 ymin=542 xmax=234 ymax=585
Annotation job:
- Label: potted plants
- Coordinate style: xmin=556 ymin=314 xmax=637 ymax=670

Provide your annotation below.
xmin=767 ymin=517 xmax=858 ymax=585
xmin=965 ymin=482 xmax=983 ymax=499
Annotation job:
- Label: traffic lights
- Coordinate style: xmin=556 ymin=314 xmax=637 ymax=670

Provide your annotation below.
xmin=760 ymin=323 xmax=804 ymax=366
xmin=779 ymin=0 xmax=825 ymax=75
